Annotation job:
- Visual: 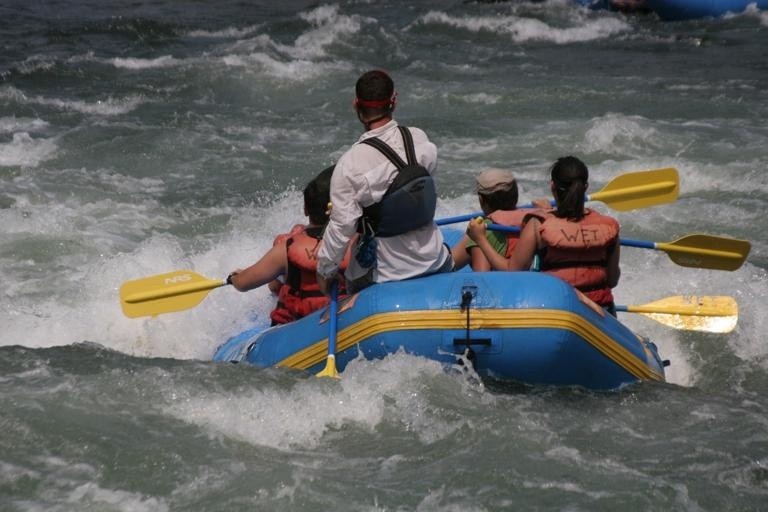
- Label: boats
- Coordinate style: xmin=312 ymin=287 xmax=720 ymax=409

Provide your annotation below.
xmin=211 ymin=226 xmax=670 ymax=390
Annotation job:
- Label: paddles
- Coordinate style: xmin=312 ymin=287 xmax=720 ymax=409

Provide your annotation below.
xmin=620 ymin=234 xmax=752 ymax=271
xmin=616 ymin=295 xmax=737 ymax=333
xmin=315 ymin=279 xmax=341 ymax=379
xmin=435 ymin=168 xmax=680 ymax=225
xmin=120 ymin=272 xmax=236 ymax=317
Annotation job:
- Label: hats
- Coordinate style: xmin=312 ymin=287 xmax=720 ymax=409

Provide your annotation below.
xmin=475 ymin=167 xmax=517 ymax=196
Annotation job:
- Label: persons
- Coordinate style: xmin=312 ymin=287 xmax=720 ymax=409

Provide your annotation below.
xmin=225 ymin=176 xmax=360 ymax=330
xmin=313 ymin=70 xmax=456 ymax=293
xmin=465 ymin=154 xmax=622 ymax=324
xmin=443 ymin=167 xmax=555 ymax=275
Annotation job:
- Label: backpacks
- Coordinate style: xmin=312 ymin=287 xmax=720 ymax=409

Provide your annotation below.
xmin=361 ymin=124 xmax=434 ymax=235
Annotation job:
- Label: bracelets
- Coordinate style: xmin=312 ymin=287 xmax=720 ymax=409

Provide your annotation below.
xmin=227 ymin=273 xmax=237 ymax=285
